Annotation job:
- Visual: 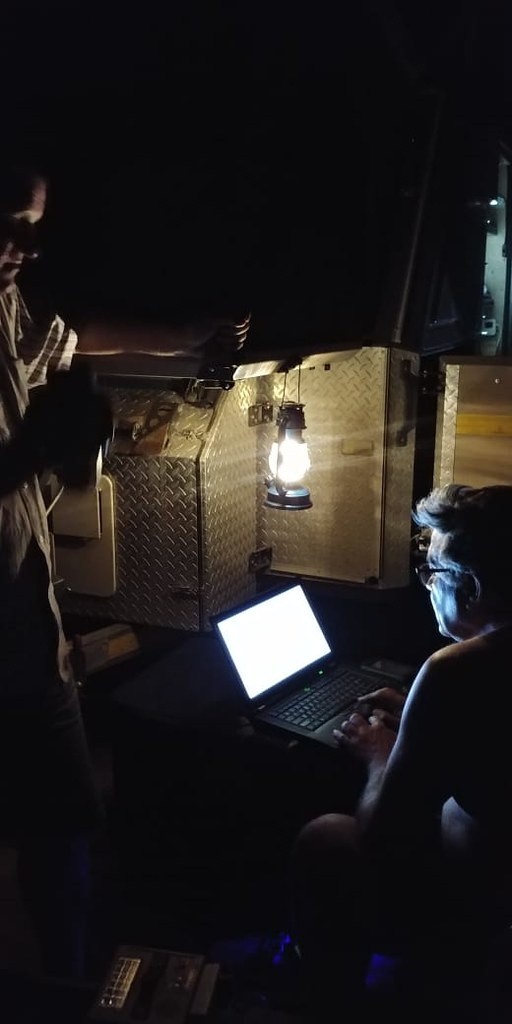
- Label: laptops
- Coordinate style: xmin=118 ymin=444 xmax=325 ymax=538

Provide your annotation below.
xmin=208 ymin=577 xmax=405 ymax=753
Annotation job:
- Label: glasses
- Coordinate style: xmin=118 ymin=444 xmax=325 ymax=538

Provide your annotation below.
xmin=416 ymin=562 xmax=476 ymax=591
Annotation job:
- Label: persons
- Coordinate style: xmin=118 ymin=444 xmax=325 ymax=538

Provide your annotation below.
xmin=249 ymin=486 xmax=512 ymax=1024
xmin=0 ymin=133 xmax=251 ymax=1024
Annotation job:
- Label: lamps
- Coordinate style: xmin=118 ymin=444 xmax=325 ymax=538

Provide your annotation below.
xmin=261 ymin=356 xmax=313 ymax=509
